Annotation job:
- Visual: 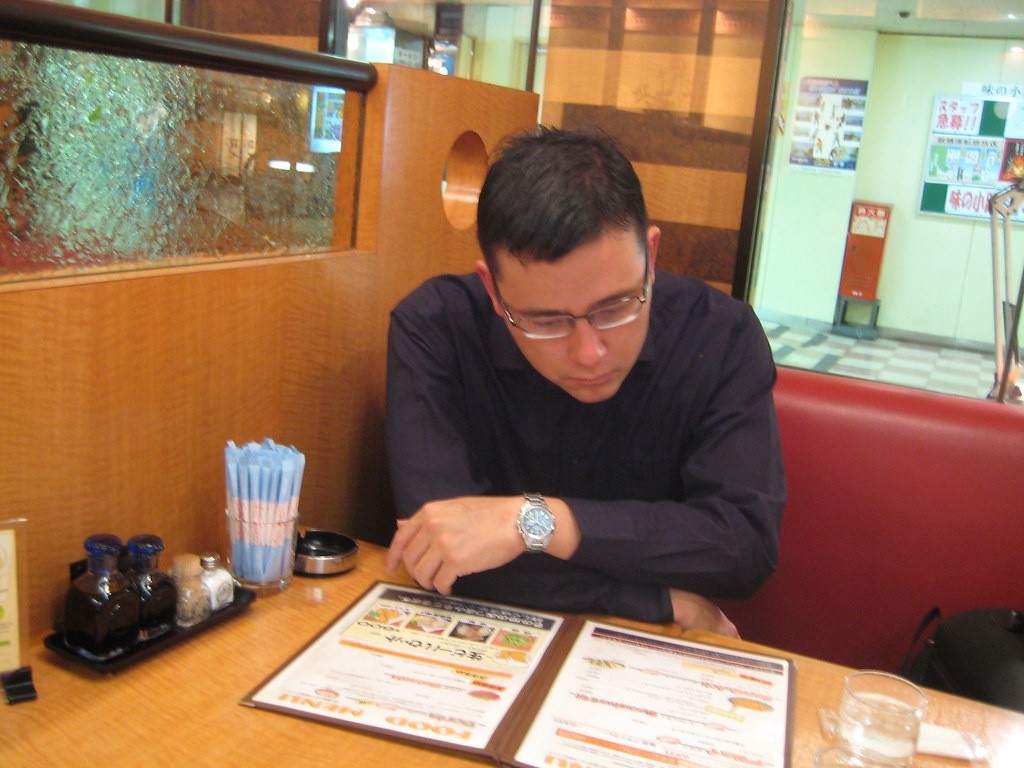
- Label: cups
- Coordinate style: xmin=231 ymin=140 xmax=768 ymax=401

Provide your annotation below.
xmin=815 ymin=745 xmax=907 ymax=768
xmin=836 ymin=670 xmax=929 ymax=764
xmin=225 ymin=506 xmax=300 ymax=592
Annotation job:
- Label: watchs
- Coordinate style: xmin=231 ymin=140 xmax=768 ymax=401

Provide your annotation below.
xmin=517 ymin=493 xmax=555 ymax=551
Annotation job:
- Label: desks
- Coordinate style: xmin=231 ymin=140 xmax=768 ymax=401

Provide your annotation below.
xmin=0 ymin=527 xmax=1023 ymax=767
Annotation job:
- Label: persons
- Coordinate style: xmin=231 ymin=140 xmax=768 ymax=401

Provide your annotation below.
xmin=383 ymin=129 xmax=787 ymax=639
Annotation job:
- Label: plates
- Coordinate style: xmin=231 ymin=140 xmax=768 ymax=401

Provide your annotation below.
xmin=417 ymin=614 xmax=450 ymax=633
xmin=371 ymin=606 xmax=407 ymax=624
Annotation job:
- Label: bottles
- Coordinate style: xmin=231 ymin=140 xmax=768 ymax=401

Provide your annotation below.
xmin=62 ymin=533 xmax=140 ymax=656
xmin=200 ymin=551 xmax=233 ymax=611
xmin=124 ymin=533 xmax=178 ymax=640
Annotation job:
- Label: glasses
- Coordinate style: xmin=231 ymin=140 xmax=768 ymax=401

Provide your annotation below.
xmin=493 ymin=247 xmax=649 ymax=340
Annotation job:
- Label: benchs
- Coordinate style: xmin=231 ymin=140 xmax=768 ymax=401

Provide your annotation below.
xmin=712 ymin=365 xmax=1023 ymax=675
